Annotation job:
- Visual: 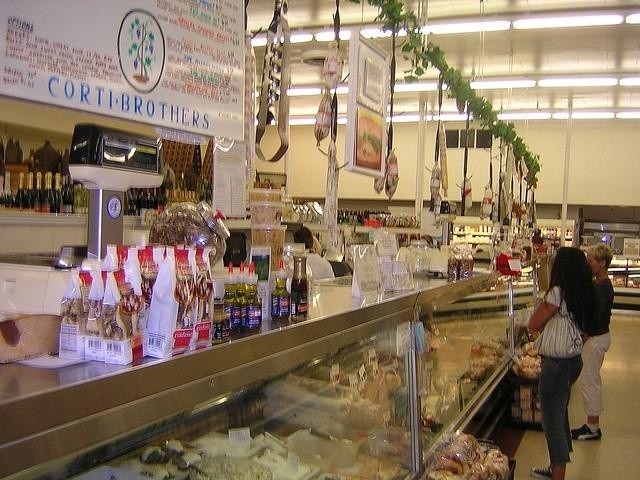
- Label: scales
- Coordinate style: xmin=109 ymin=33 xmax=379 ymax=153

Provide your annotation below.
xmin=65 ymin=123 xmax=167 ymax=188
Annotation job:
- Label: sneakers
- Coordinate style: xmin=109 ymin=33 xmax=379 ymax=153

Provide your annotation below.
xmin=571 ymin=424 xmax=601 ymax=440
xmin=530 ymin=467 xmax=552 ymax=480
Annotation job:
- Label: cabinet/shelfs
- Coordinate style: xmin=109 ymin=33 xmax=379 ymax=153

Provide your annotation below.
xmin=282 ymin=212 xmax=579 ymax=276
xmin=579 ymin=222 xmax=640 ymax=310
xmin=414 ymin=267 xmax=511 ymax=479
xmin=511 ymin=260 xmax=536 ymax=362
xmin=0 ymin=293 xmax=414 ymax=480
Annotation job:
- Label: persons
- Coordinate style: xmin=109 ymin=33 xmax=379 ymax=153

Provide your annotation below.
xmin=291 ymin=227 xmax=335 ymax=280
xmin=570 ymin=242 xmax=614 ymax=441
xmin=528 ymin=247 xmax=595 ymax=480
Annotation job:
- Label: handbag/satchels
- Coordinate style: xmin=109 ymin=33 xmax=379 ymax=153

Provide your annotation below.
xmin=535 ymin=299 xmax=583 ymax=359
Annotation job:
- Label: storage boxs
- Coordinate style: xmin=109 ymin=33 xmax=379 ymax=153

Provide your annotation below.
xmin=251 ymin=202 xmax=286 ymax=225
xmin=252 ymin=225 xmax=287 ymax=270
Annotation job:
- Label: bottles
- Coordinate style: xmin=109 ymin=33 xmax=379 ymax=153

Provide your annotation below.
xmin=289 ymin=255 xmax=309 ymax=322
xmin=404 ymin=238 xmax=431 ymax=291
xmin=211 ymin=260 xmax=262 ymax=345
xmin=121 ymin=169 xmax=211 ymax=218
xmin=270 ymin=259 xmax=292 ymax=320
xmin=0 ymin=170 xmax=88 ymax=215
